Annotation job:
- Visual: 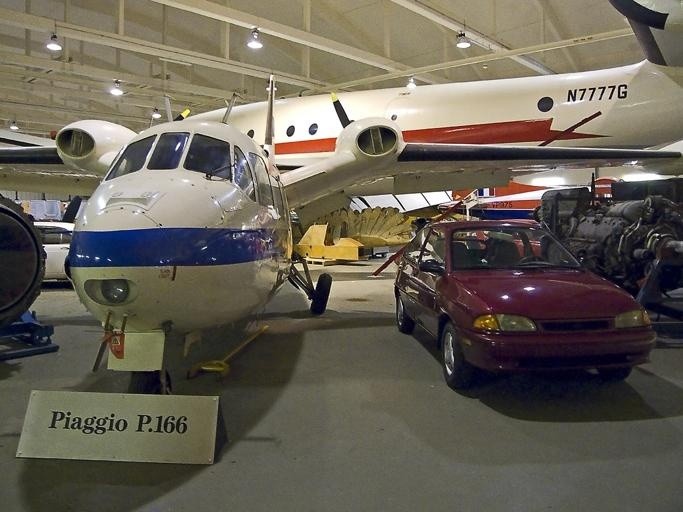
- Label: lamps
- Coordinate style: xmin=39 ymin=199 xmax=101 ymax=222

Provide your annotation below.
xmin=455 ymin=28 xmax=471 ymax=50
xmin=46 ymin=32 xmax=62 ymax=52
xmin=9 ymin=120 xmax=19 ymax=130
xmin=151 ymin=108 xmax=162 ymax=119
xmin=110 ymin=79 xmax=124 ymax=96
xmin=406 ymin=76 xmax=417 ymax=90
xmin=245 ymin=28 xmax=264 ymax=50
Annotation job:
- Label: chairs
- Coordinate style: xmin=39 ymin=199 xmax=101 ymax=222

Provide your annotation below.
xmin=450 ymin=241 xmax=475 ymax=270
xmin=493 ymin=239 xmax=521 ymax=270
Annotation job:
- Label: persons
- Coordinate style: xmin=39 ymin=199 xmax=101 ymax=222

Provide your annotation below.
xmin=59 ymin=201 xmax=67 ymax=218
